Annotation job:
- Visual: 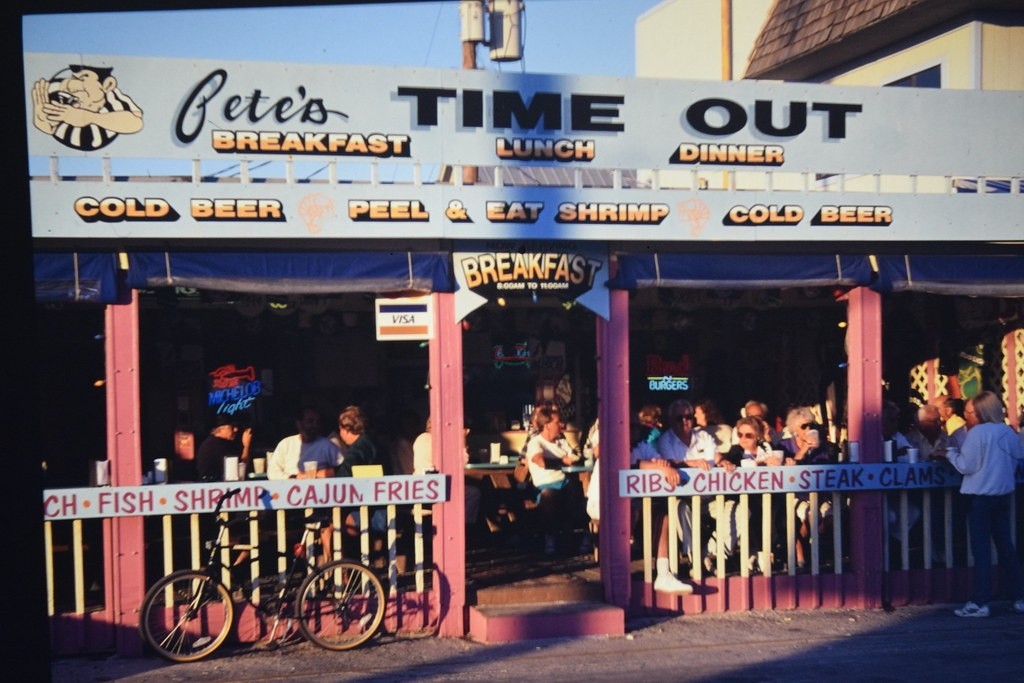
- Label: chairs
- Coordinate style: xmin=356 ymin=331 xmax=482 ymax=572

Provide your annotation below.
xmin=388 ymin=439 xmax=414 ymax=475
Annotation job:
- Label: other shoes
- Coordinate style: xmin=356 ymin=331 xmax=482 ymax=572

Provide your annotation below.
xmin=654 ymin=573 xmax=693 ymax=594
xmin=690 ymin=555 xmax=801 ymax=577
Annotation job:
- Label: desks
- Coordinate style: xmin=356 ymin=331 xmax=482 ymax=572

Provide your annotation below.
xmin=466 ymin=468 xmax=533 ymax=549
xmin=563 ymin=465 xmax=593 ymax=499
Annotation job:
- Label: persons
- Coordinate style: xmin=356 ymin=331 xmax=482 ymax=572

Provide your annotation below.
xmin=196 ymin=403 xmax=491 ymax=599
xmin=659 ymin=398 xmax=848 ymax=574
xmin=883 ymin=391 xmax=1024 ymax=618
xmin=516 ymin=402 xmax=693 ymax=594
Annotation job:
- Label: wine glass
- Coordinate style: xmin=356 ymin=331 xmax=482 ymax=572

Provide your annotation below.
xmin=523 ymin=405 xmax=535 ymax=432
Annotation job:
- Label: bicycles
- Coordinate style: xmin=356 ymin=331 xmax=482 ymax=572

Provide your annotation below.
xmin=133 ymin=486 xmax=389 ymax=663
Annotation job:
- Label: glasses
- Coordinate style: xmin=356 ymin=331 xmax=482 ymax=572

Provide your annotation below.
xmin=673 ymin=414 xmax=693 ymax=422
xmin=738 ymin=432 xmax=757 ymax=439
xmin=799 ymin=422 xmax=815 ymax=430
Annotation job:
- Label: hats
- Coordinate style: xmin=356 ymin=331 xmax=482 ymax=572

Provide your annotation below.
xmin=212 ymin=414 xmax=237 ymax=428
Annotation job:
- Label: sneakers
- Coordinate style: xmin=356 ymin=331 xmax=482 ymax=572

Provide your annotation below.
xmin=954 ymin=600 xmax=990 ymax=618
xmin=1008 ymin=601 xmax=1024 ymax=612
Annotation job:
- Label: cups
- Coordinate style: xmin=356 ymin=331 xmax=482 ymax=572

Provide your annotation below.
xmin=511 ymin=420 xmax=520 ymax=430
xmin=807 ymin=430 xmax=818 ymax=447
xmin=946 ymin=447 xmax=959 ymax=455
xmin=253 ymin=458 xmax=266 ymax=474
xmin=772 ymin=450 xmax=784 ymax=465
xmin=239 ymin=463 xmax=246 ymax=481
xmin=907 ymin=448 xmax=918 ymax=464
xmin=304 ymin=461 xmax=317 ymax=480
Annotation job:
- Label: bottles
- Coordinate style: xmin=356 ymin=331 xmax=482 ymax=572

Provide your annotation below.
xmin=583 ymin=444 xmax=593 ymax=466
